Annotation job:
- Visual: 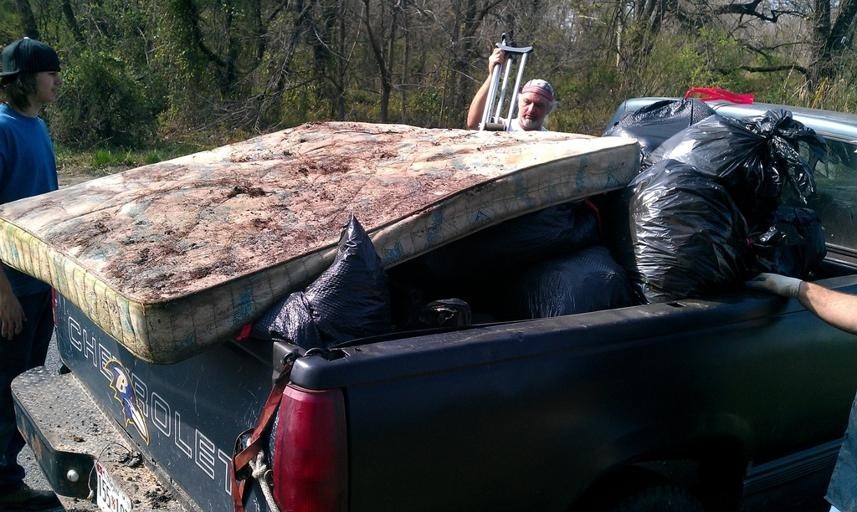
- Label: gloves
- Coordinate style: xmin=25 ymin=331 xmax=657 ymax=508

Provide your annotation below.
xmin=746 ymin=272 xmax=803 ymax=299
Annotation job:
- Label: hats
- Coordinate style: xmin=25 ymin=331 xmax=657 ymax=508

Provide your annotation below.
xmin=0 ymin=36 xmax=61 ymax=78
xmin=520 ymin=78 xmax=554 ymax=101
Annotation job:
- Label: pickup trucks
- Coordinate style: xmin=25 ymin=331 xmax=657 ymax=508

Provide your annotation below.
xmin=10 ymin=91 xmax=857 ymax=511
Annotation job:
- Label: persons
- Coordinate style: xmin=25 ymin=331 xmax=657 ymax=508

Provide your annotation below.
xmin=1 ymin=36 xmax=63 ymax=512
xmin=466 ymin=47 xmax=553 ymax=133
xmin=743 ymin=271 xmax=856 ymax=512
